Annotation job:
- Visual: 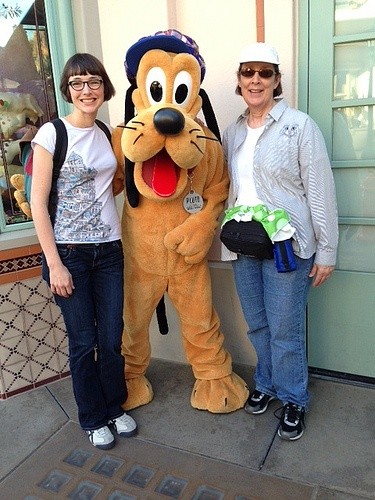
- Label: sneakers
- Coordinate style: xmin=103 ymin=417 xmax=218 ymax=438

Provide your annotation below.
xmin=108 ymin=413 xmax=136 ymax=438
xmin=86 ymin=425 xmax=115 ymax=449
xmin=274 ymin=401 xmax=303 ymax=441
xmin=243 ymin=390 xmax=274 ymax=415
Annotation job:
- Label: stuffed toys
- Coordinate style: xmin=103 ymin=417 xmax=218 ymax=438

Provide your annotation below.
xmin=1 ymin=112 xmax=27 ymax=165
xmin=10 ymin=29 xmax=251 ymax=414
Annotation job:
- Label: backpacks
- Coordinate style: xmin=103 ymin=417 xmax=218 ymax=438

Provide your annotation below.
xmin=22 ymin=118 xmax=112 ymax=218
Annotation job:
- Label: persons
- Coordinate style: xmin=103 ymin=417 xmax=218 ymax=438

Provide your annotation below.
xmin=220 ymin=43 xmax=339 ymax=441
xmin=29 ymin=52 xmax=139 ymax=452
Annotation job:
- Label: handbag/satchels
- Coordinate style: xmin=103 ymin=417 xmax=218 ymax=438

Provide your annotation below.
xmin=220 ymin=217 xmax=272 ymax=259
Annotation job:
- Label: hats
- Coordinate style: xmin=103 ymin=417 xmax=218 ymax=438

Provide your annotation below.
xmin=239 ymin=42 xmax=279 ymax=65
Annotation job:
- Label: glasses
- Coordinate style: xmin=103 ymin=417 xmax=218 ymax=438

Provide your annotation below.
xmin=240 ymin=67 xmax=278 ymax=78
xmin=68 ymin=79 xmax=103 ymax=91
